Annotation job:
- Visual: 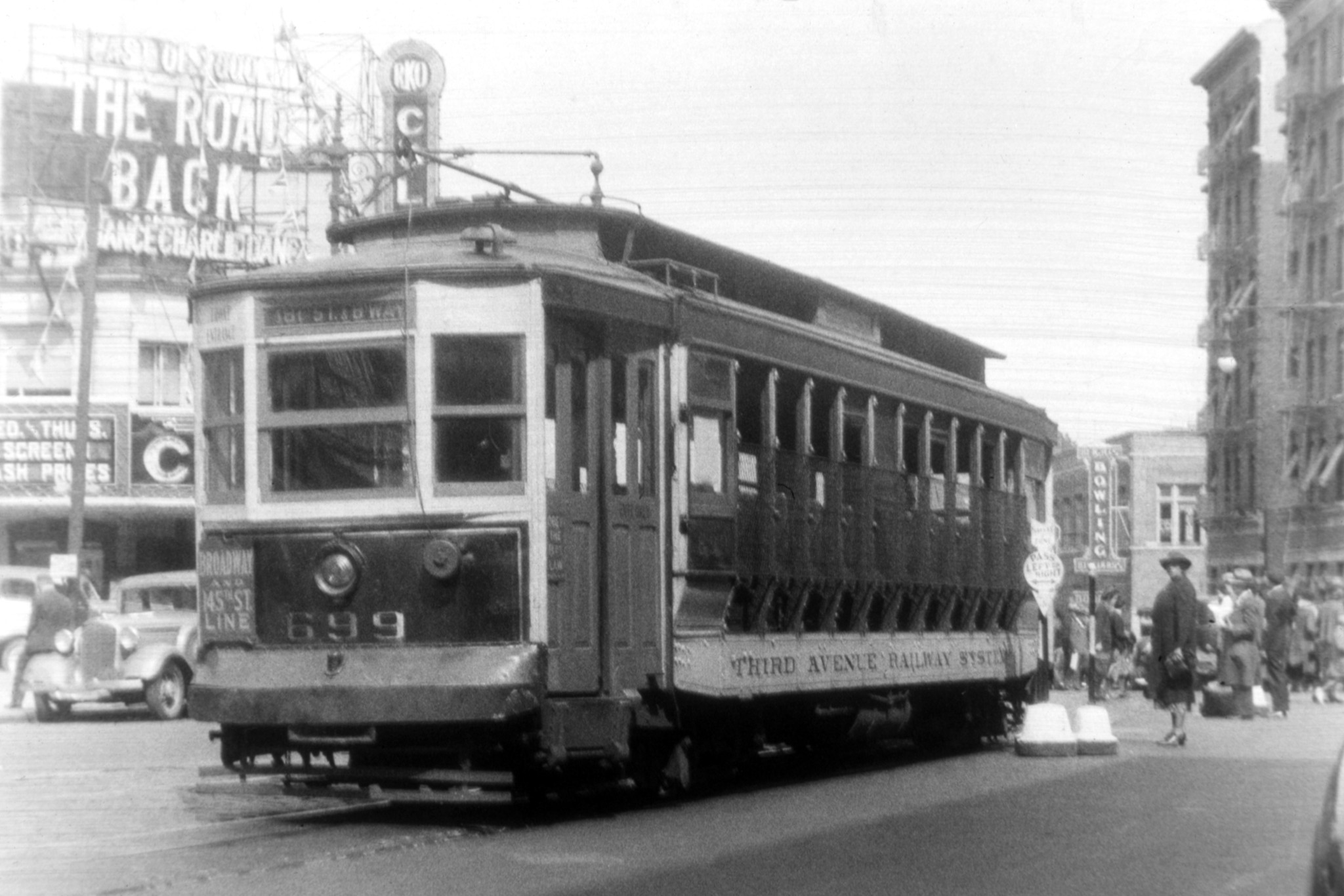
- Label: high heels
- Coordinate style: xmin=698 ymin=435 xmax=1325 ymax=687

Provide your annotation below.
xmin=1160 ymin=731 xmax=1186 ymax=746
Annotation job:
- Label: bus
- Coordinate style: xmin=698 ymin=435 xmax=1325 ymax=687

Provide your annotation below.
xmin=187 ymin=116 xmax=1061 ymax=822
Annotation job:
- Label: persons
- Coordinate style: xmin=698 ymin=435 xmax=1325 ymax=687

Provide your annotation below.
xmin=1261 ymin=565 xmax=1296 ymax=715
xmin=1150 ymin=550 xmax=1198 ymax=747
xmin=1052 ymin=568 xmax=1344 ymax=707
xmin=60 ymin=576 xmax=88 ymax=625
xmin=1217 ymin=567 xmax=1261 ymax=720
xmin=8 ymin=574 xmax=76 ymax=708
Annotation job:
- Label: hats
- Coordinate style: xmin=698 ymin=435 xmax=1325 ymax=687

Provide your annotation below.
xmin=1158 ymin=550 xmax=1192 ymax=571
xmin=1265 ymin=564 xmax=1285 ymax=583
xmin=1226 ymin=569 xmax=1253 ymax=586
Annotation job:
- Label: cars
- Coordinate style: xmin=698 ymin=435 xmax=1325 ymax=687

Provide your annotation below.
xmin=25 ymin=570 xmax=206 ymax=722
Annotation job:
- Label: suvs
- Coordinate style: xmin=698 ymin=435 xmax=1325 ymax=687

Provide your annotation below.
xmin=0 ymin=565 xmax=102 ymax=679
